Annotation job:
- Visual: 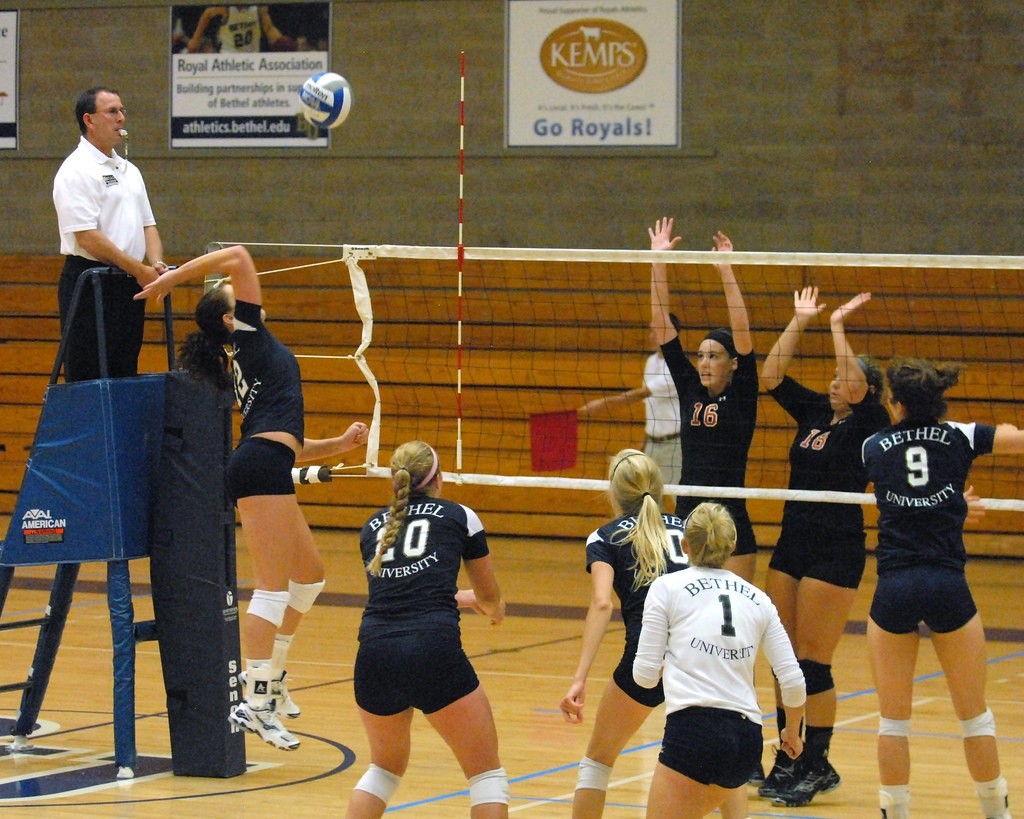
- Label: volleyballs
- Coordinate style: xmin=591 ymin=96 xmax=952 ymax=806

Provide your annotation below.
xmin=299 ymin=72 xmax=354 ymax=129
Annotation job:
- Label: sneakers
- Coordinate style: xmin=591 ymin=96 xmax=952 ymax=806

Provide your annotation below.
xmin=758 ymin=745 xmax=803 ymax=798
xmin=771 ymin=758 xmax=840 ymax=808
xmin=237 ymin=670 xmax=300 ymax=720
xmin=227 ymin=698 xmax=300 ymax=752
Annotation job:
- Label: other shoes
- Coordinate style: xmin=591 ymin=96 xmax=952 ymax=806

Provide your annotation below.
xmin=748 ymin=763 xmax=765 ymax=786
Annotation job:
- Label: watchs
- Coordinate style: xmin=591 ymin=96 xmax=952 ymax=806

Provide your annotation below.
xmin=151 ymin=259 xmax=168 ymax=269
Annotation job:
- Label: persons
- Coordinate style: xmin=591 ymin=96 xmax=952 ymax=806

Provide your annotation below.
xmin=135 ymin=242 xmax=368 ymax=754
xmin=634 ymin=502 xmax=807 ymax=818
xmin=861 ymin=359 xmax=1023 ymax=819
xmin=186 ymin=1 xmax=308 ymax=54
xmin=576 ymin=312 xmax=683 ymax=515
xmin=646 ymin=217 xmax=758 ymax=588
xmin=53 ymin=87 xmax=167 ymax=383
xmin=561 ymin=449 xmax=749 ymax=819
xmin=760 ymin=287 xmax=892 ymax=655
xmin=349 ymin=441 xmax=511 ymax=818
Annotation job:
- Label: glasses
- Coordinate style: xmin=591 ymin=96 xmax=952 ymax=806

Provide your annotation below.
xmin=89 ymin=108 xmax=126 ymax=116
